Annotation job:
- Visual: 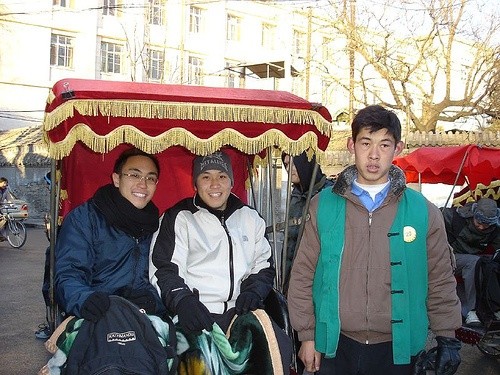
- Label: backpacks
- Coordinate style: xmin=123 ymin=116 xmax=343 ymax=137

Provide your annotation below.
xmin=60 ymin=295 xmax=171 ymax=375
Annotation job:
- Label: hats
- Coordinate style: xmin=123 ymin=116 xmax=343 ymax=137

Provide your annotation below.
xmin=456 ymin=198 xmax=500 ymax=226
xmin=192 ymin=150 xmax=234 ymax=187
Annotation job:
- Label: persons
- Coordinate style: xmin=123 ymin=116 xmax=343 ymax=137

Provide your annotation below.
xmin=439 ymin=198 xmax=499 ymax=326
xmin=0 ymin=177 xmax=14 ymax=202
xmin=148 ymin=151 xmax=277 ymax=374
xmin=281 ymin=147 xmax=337 ymax=292
xmin=286 ymin=105 xmax=460 ymax=375
xmin=52 ymin=149 xmax=179 ymax=374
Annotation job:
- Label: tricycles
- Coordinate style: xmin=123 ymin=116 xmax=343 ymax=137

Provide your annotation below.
xmin=0 ymin=204 xmax=28 ymax=250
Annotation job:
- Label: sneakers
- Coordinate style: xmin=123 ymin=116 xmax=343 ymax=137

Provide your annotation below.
xmin=465 ymin=310 xmax=481 ymax=328
xmin=35 ymin=326 xmax=50 ymax=339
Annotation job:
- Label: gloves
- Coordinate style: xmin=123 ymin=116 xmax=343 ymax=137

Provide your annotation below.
xmin=129 ymin=288 xmax=155 ymax=310
xmin=434 ymin=336 xmax=462 ymax=375
xmin=177 ymin=288 xmax=215 ymax=336
xmin=235 ymin=292 xmax=266 ymax=316
xmin=80 ymin=292 xmax=111 ymax=322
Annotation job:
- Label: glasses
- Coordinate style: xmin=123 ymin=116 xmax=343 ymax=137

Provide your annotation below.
xmin=120 ymin=173 xmax=159 ymax=185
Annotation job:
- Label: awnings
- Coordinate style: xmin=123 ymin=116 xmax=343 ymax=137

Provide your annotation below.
xmin=43 ymin=77 xmax=332 ymax=374
xmin=391 ymin=145 xmax=500 ymax=208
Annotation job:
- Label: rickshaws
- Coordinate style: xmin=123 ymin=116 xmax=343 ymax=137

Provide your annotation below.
xmin=393 ymin=144 xmax=500 ymax=375
xmin=40 ymin=81 xmax=332 ymax=375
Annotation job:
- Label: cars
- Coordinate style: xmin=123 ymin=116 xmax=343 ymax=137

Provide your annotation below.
xmin=0 ymin=190 xmax=28 ymax=228
